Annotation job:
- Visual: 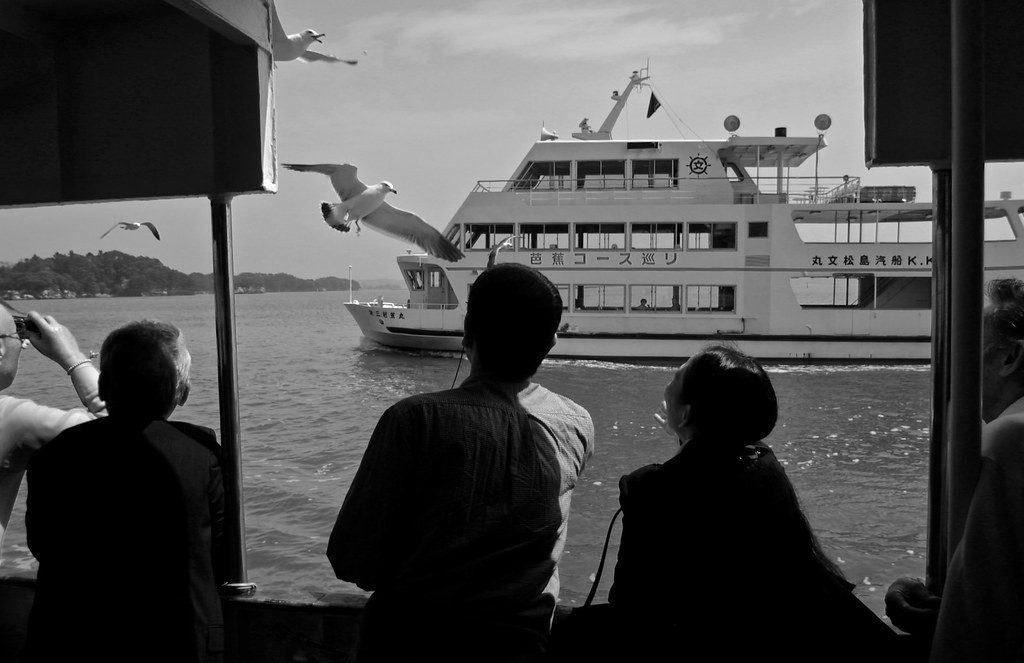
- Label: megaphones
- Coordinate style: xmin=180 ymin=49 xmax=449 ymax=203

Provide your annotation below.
xmin=724 ymin=116 xmax=740 ymax=132
xmin=814 ymin=114 xmax=830 ymax=131
xmin=540 ymin=128 xmax=558 ymax=141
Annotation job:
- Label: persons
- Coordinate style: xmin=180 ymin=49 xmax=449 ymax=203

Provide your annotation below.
xmin=326 ymin=265 xmax=594 ymax=663
xmin=884 ymin=278 xmax=1024 ymax=663
xmin=607 ymin=346 xmax=929 ymax=663
xmin=638 ymin=299 xmax=647 ymax=308
xmin=669 ymin=297 xmax=680 ymax=311
xmin=25 ymin=320 xmax=240 ymax=663
xmin=0 ymin=295 xmax=109 ymax=552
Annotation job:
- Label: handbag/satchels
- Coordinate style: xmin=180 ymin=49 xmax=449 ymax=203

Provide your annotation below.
xmin=551 ymin=465 xmax=676 ymax=663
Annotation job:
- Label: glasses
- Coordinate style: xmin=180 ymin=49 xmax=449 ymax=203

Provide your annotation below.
xmin=0 ymin=321 xmax=28 ymax=339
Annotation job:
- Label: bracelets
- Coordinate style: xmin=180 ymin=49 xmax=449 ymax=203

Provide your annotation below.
xmin=66 ymin=359 xmax=91 ymax=375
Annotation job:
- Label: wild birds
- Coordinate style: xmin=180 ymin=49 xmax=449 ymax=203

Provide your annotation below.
xmin=100 ymin=221 xmax=160 ymax=241
xmin=272 ymin=0 xmax=359 ymax=66
xmin=282 ymin=162 xmax=467 ymax=263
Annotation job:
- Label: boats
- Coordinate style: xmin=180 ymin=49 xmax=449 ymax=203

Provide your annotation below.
xmin=344 ymin=57 xmax=1024 ymax=366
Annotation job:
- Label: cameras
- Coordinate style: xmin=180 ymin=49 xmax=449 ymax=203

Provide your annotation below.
xmin=12 ymin=315 xmax=40 ymax=339
